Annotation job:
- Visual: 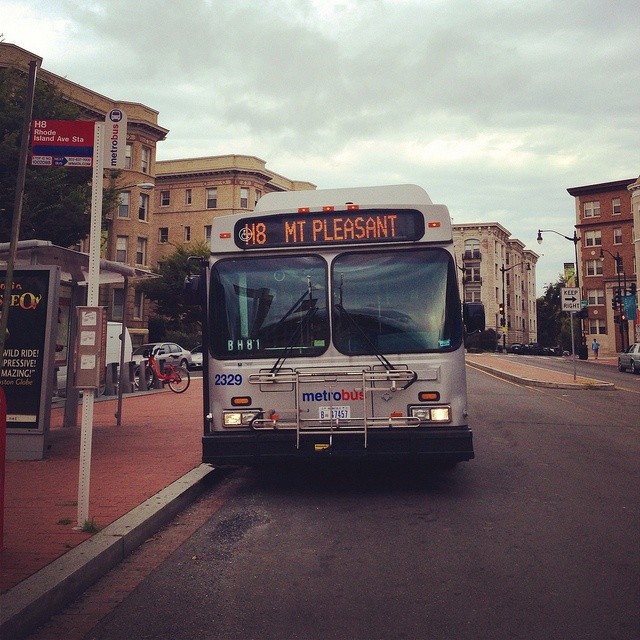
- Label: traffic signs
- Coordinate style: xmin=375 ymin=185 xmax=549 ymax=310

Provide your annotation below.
xmin=561 ymin=288 xmax=582 ymax=312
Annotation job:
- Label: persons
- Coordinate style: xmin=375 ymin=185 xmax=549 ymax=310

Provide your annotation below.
xmin=592 ymin=339 xmax=600 ymax=360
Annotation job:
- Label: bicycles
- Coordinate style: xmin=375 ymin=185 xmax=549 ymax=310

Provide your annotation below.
xmin=134 ymin=347 xmax=190 ymax=393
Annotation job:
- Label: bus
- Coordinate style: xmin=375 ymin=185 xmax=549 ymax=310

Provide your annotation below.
xmin=185 ymin=184 xmax=485 ymax=460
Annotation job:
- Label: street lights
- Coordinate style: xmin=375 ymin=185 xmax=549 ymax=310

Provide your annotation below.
xmin=599 ymin=249 xmax=624 ymax=352
xmin=537 ymin=229 xmax=581 ymax=343
xmin=499 ymin=261 xmax=531 ymax=355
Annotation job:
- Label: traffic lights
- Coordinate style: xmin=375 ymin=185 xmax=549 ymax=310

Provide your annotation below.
xmin=614 ymin=316 xmax=620 ymax=324
xmin=499 ymin=304 xmax=504 ymax=314
xmin=629 ymin=283 xmax=636 ymax=294
xmin=612 ymin=299 xmax=616 ymax=310
xmin=621 ymin=314 xmax=626 ymax=320
xmin=615 ymin=289 xmax=621 ymax=303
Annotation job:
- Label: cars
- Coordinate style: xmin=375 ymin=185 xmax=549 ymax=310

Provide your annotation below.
xmin=132 ymin=343 xmax=192 ymax=371
xmin=618 ymin=343 xmax=640 ymax=373
xmin=189 ymin=344 xmax=204 ymax=367
